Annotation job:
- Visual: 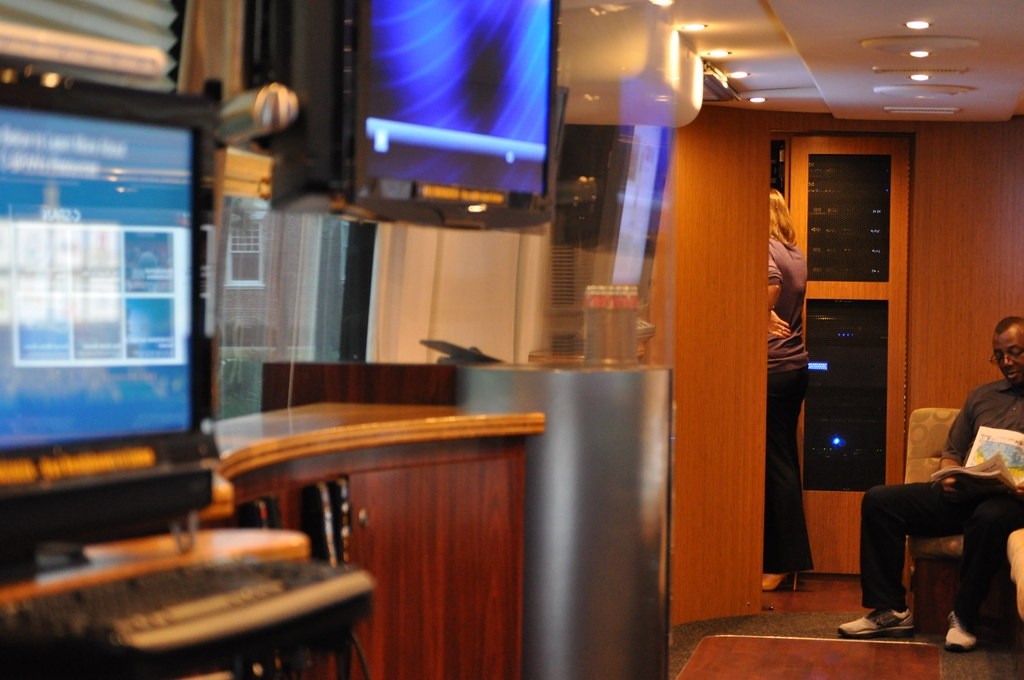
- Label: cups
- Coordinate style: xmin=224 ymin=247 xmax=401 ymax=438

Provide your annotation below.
xmin=582 ymin=283 xmax=643 ymax=366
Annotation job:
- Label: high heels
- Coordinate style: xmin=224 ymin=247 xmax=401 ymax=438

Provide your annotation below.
xmin=762 ymin=571 xmax=797 ymax=591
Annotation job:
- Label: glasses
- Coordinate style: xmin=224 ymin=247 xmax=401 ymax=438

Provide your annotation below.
xmin=990 ymin=349 xmax=1024 ymax=365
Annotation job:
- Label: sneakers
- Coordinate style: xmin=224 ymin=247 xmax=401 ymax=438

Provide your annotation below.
xmin=943 ymin=611 xmax=977 ymax=652
xmin=837 ymin=607 xmax=915 ymax=638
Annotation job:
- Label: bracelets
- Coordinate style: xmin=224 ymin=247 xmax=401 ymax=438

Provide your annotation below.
xmin=768 ymin=306 xmax=774 ymax=311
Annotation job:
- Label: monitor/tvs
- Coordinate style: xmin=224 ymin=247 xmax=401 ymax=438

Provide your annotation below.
xmin=0 ymin=70 xmax=223 ymax=557
xmin=269 ymin=0 xmax=561 ymax=230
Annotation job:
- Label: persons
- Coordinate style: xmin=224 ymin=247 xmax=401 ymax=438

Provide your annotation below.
xmin=837 ymin=316 xmax=1024 ymax=651
xmin=762 ymin=188 xmax=813 ymax=590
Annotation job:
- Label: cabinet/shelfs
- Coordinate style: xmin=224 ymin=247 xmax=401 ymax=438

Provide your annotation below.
xmin=207 ymin=431 xmax=525 ymax=680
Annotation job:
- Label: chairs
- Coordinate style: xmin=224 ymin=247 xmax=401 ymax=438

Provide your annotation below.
xmin=904 ymin=407 xmax=1010 ymax=635
